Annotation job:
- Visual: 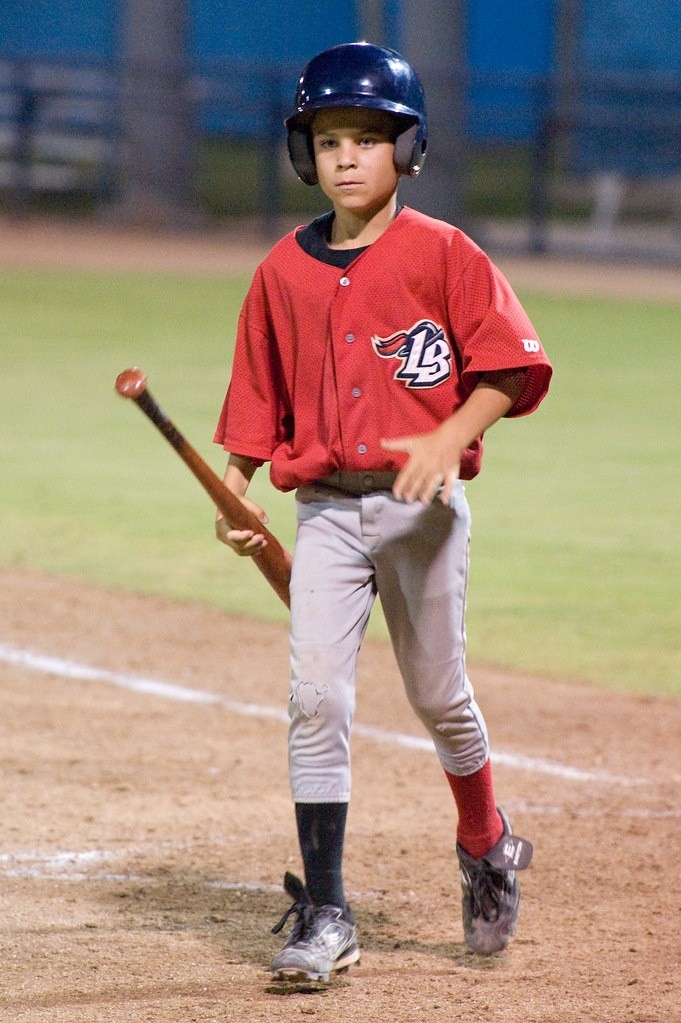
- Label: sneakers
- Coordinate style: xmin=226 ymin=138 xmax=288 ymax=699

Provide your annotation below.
xmin=454 ymin=807 xmax=533 ymax=955
xmin=271 ymin=871 xmax=361 ymax=980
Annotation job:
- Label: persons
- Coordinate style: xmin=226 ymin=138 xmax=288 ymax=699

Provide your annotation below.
xmin=211 ymin=40 xmax=556 ymax=996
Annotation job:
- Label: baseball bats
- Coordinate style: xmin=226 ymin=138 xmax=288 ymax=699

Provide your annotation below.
xmin=114 ymin=367 xmax=293 ymax=611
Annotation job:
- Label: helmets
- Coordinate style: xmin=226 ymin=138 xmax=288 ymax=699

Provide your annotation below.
xmin=283 ymin=42 xmax=428 ymax=186
